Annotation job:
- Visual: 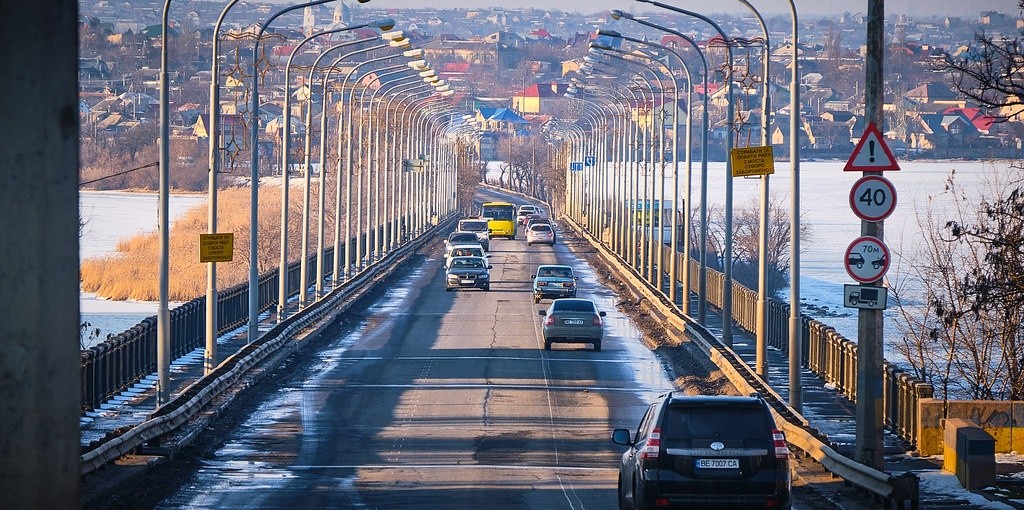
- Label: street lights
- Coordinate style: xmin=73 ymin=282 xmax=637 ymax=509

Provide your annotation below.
xmin=275 ymin=18 xmax=393 ymax=324
xmin=301 ymin=30 xmax=485 ymax=312
xmin=562 ymin=9 xmax=692 ymax=312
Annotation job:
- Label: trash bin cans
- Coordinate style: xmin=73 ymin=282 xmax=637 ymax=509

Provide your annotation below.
xmin=944 ymin=417 xmax=996 ymax=491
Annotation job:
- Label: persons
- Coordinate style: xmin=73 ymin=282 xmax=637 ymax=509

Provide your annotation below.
xmin=457 ymin=249 xmax=465 ymax=256
xmin=471 ymin=250 xmax=478 ymax=256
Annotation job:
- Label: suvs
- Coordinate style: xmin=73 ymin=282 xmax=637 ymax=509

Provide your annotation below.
xmin=610 ymin=389 xmax=792 ymax=509
xmin=444 ymin=233 xmax=484 ymax=257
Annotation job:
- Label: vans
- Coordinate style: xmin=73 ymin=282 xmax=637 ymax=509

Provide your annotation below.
xmin=456 ymin=219 xmax=491 ymax=252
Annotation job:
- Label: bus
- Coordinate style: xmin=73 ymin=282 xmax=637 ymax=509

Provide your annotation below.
xmin=481 ymin=202 xmax=517 ymax=239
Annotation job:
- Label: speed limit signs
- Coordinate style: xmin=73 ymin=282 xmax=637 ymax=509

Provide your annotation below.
xmin=849 ymin=177 xmax=899 ymax=223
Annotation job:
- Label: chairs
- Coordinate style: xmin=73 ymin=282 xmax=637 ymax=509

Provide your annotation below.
xmin=456 ymin=262 xmax=463 ymax=268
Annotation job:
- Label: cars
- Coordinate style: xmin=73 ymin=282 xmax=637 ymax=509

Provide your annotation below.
xmin=517 ymin=205 xmax=553 ymax=236
xmin=531 ymin=264 xmax=579 ymax=304
xmin=527 ymin=224 xmax=555 ymax=247
xmin=443 ymin=244 xmax=492 ymax=272
xmin=443 ymin=256 xmax=492 ymax=291
xmin=539 ymin=299 xmax=606 ymax=350
